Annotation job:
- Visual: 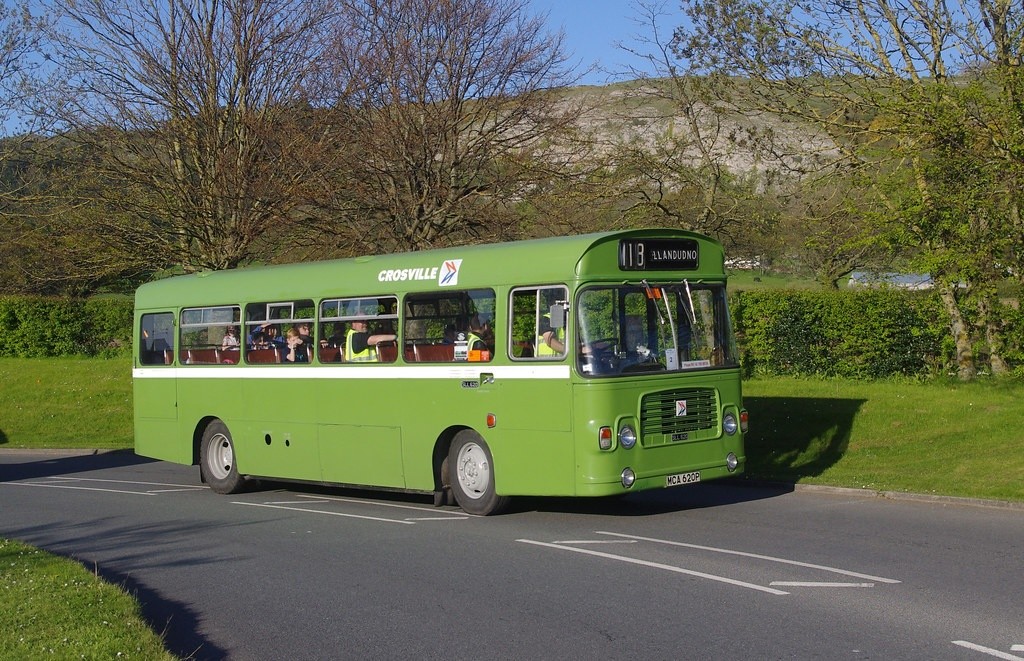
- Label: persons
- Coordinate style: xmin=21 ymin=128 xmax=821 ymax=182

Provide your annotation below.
xmin=538 ymin=291 xmax=592 ymax=357
xmin=441 ymin=315 xmax=494 ymax=362
xmin=328 ymin=312 xmax=397 ymax=363
xmin=222 ymin=324 xmax=329 ymax=362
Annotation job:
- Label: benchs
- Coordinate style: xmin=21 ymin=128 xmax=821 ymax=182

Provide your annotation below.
xmin=140 ymin=337 xmax=454 ymax=365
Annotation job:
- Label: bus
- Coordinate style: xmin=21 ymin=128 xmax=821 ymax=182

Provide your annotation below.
xmin=132 ymin=227 xmax=746 ymax=516
